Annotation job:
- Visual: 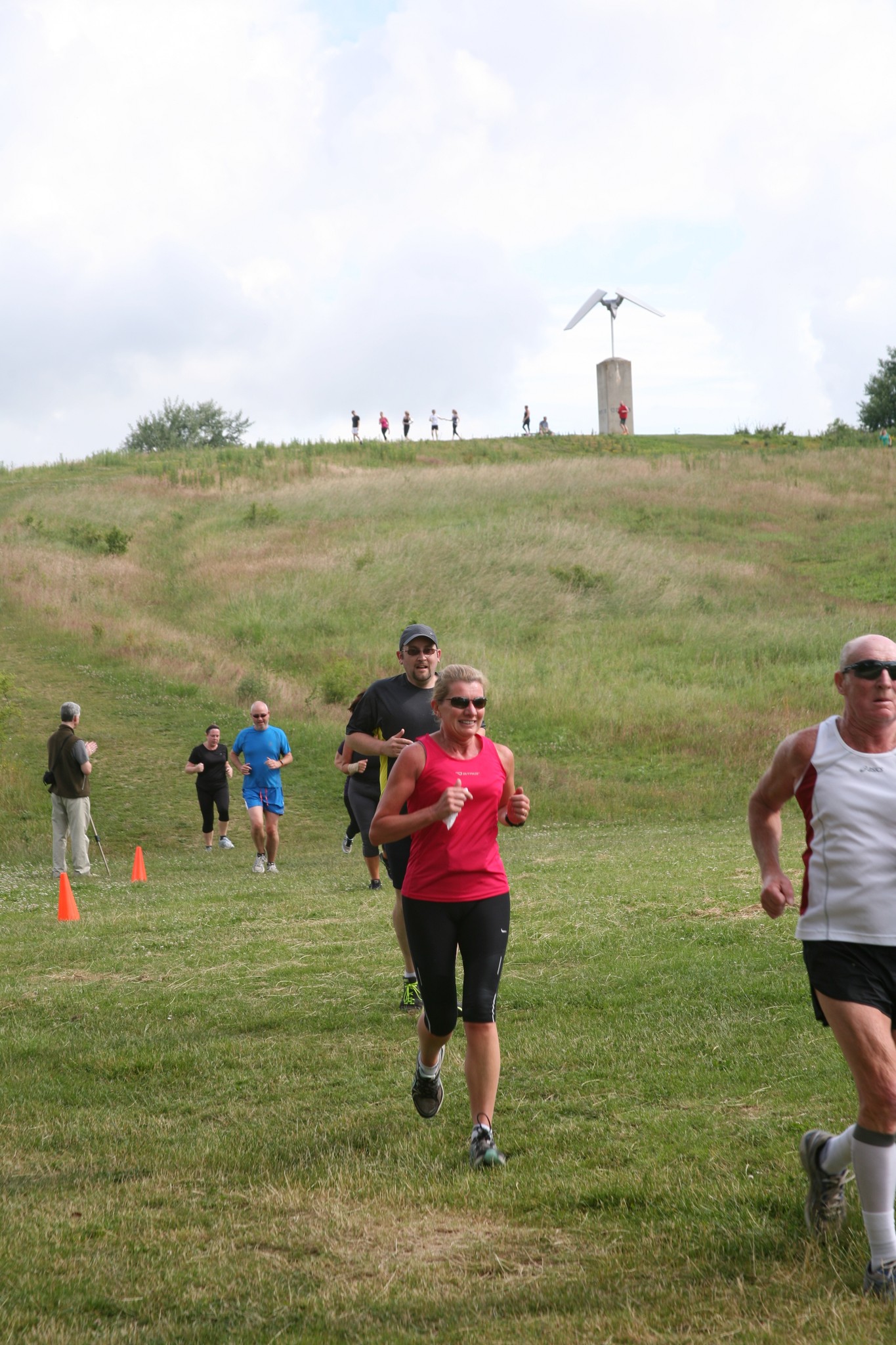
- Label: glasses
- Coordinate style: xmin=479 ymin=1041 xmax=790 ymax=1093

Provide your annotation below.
xmin=401 ymin=648 xmax=438 ymax=655
xmin=251 ymin=713 xmax=268 ymax=718
xmin=842 ymin=659 xmax=896 ymax=682
xmin=437 ymin=697 xmax=489 ymax=709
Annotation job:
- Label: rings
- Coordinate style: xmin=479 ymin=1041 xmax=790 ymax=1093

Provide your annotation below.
xmin=524 ymin=810 xmax=527 ymax=816
xmin=92 ymin=750 xmax=93 ymax=752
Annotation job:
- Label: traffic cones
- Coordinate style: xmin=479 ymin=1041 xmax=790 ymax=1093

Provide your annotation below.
xmin=131 ymin=846 xmax=148 ymax=883
xmin=57 ymin=872 xmax=80 ymax=921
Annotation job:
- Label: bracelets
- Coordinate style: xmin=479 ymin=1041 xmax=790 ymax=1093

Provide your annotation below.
xmin=279 ymin=759 xmax=284 ymax=767
xmin=505 ymin=813 xmax=525 ymax=827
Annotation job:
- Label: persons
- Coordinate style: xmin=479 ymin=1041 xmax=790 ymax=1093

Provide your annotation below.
xmin=750 ymin=634 xmax=896 ymax=1294
xmin=341 ymin=691 xmax=393 ymax=890
xmin=345 ymin=624 xmax=485 ymax=1017
xmin=42 ymin=702 xmax=100 ymax=879
xmin=229 ymin=701 xmax=292 ymax=873
xmin=368 ymin=663 xmax=530 ymax=1167
xmin=334 ymin=740 xmax=360 ymax=853
xmin=185 ymin=725 xmax=234 ymax=852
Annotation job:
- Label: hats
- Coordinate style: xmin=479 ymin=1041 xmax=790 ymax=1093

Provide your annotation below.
xmin=399 ymin=624 xmax=439 ymax=651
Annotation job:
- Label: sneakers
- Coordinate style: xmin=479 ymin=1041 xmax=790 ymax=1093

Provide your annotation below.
xmin=799 ymin=1128 xmax=854 ymax=1240
xmin=863 ymin=1260 xmax=896 ymax=1306
xmin=252 ymin=847 xmax=267 ymax=873
xmin=379 ymin=849 xmax=393 ymax=881
xmin=266 ymin=861 xmax=280 ymax=874
xmin=369 ymin=878 xmax=383 ymax=891
xmin=469 ymin=1130 xmax=507 ymax=1171
xmin=399 ymin=975 xmax=423 ymax=1019
xmin=411 ymin=1044 xmax=445 ymax=1119
xmin=218 ymin=837 xmax=235 ymax=850
xmin=342 ymin=832 xmax=354 ymax=854
xmin=204 ymin=847 xmax=212 ymax=854
xmin=457 ymin=997 xmax=463 ymax=1018
